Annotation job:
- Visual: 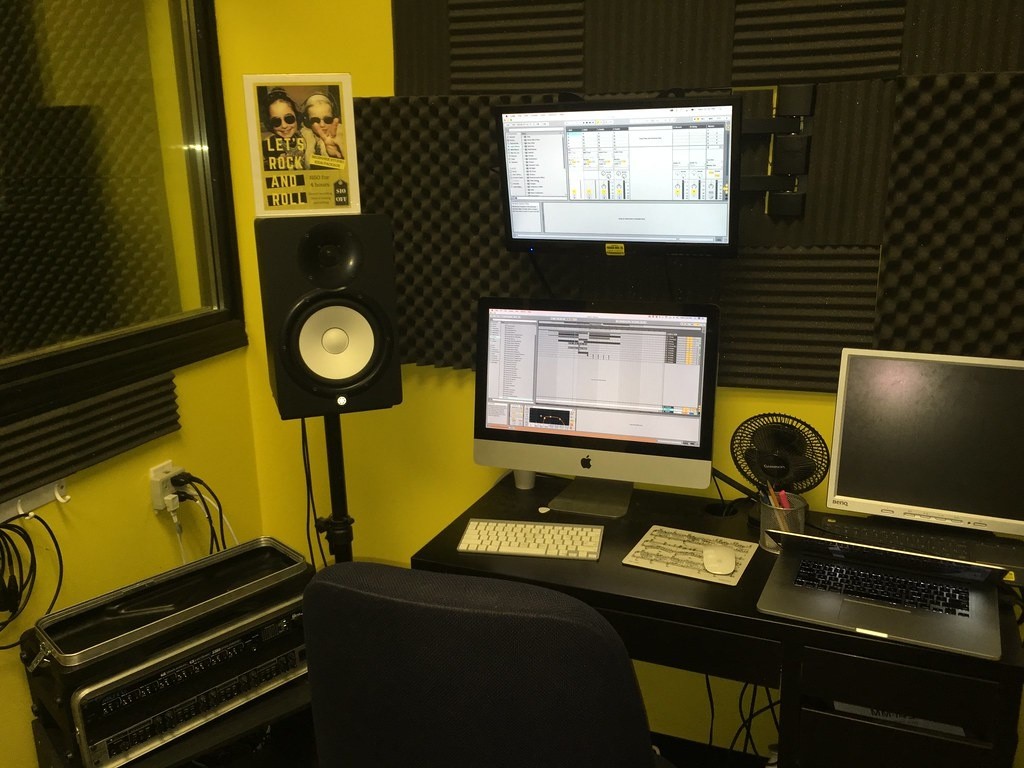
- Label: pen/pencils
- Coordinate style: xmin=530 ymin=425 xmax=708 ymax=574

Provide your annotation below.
xmin=758 ymin=479 xmax=791 ymax=532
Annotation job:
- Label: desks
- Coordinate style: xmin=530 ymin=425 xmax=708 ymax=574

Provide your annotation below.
xmin=32 ymin=672 xmax=313 ymax=768
xmin=410 ymin=472 xmax=1024 ymax=768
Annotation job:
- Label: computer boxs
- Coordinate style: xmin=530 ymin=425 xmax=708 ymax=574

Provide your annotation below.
xmin=795 ymin=663 xmax=1008 ymax=768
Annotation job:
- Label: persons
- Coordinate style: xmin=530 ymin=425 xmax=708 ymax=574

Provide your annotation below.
xmin=259 ymin=87 xmax=308 ymax=206
xmin=297 ymin=93 xmax=345 ymax=169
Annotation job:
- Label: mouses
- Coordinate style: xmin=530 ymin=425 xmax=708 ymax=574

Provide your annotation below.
xmin=701 ymin=545 xmax=735 ymax=574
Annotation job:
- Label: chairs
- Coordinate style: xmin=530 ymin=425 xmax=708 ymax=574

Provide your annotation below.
xmin=303 ymin=559 xmax=674 ymax=768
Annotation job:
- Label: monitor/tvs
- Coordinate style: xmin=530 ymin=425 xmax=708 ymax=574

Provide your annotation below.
xmin=498 ymin=95 xmax=744 ymax=253
xmin=826 ymin=348 xmax=1024 ymax=539
xmin=474 ymin=297 xmax=722 ymax=517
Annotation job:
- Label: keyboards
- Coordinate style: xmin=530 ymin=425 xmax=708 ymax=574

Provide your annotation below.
xmin=457 ymin=518 xmax=605 ymax=562
xmin=820 ymin=514 xmax=1024 ymax=587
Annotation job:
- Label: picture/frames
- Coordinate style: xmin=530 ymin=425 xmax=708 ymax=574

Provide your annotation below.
xmin=242 ymin=73 xmax=361 ymax=218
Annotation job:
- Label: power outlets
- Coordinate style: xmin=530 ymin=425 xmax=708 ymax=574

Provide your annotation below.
xmin=149 ymin=459 xmax=190 ymax=515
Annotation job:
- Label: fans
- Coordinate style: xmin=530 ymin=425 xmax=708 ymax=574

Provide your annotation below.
xmin=730 ymin=413 xmax=830 ymax=527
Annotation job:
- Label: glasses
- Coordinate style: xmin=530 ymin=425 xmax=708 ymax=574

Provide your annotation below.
xmin=310 ymin=116 xmax=333 ymax=125
xmin=271 ymin=113 xmax=295 ymax=127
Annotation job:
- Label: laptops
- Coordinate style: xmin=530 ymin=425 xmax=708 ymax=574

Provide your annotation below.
xmin=755 ymin=528 xmax=1009 ymax=662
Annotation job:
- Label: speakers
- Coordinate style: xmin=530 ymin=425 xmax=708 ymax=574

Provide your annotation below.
xmin=255 ymin=211 xmax=404 ymax=420
